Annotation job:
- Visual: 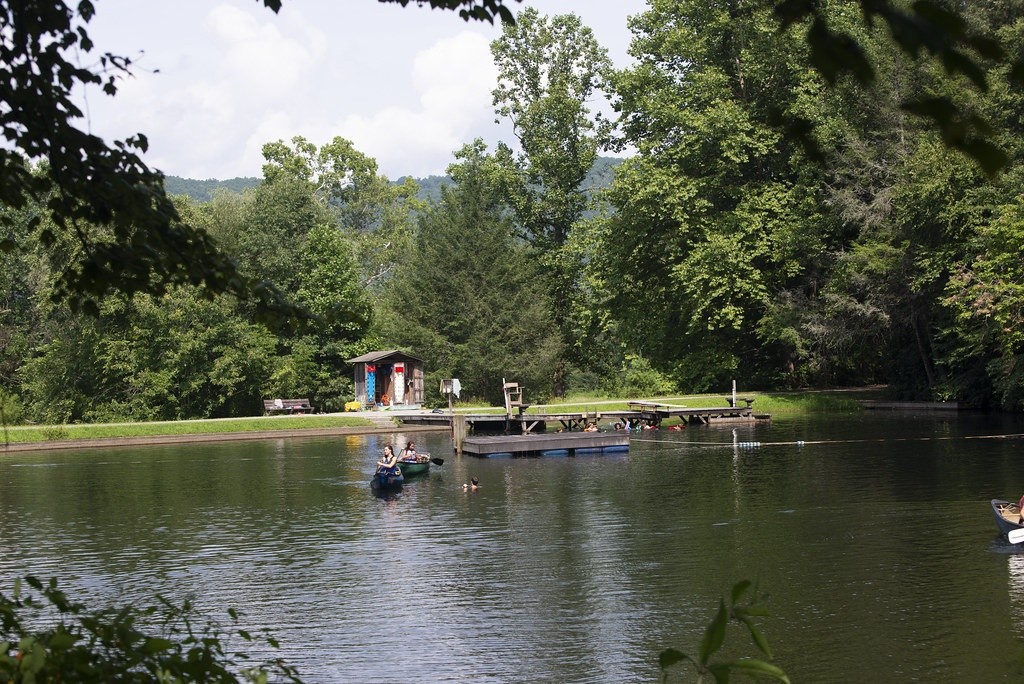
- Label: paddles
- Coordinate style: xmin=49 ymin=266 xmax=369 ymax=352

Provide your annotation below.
xmin=401 ymin=456 xmax=445 ymax=466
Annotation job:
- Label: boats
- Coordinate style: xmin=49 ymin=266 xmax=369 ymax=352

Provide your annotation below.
xmin=584 ymin=427 xmax=598 ymax=433
xmin=370 ymin=464 xmax=403 ymax=491
xmin=395 ymin=452 xmax=430 ymax=477
xmin=991 ymin=497 xmax=1024 ymax=545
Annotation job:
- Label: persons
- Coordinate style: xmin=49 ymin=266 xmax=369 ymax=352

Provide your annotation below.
xmin=588 ymin=423 xmax=594 ymax=431
xmin=677 ymin=424 xmax=683 ymax=430
xmin=400 ymin=442 xmax=424 ymax=463
xmin=615 ymin=420 xmax=652 ymax=431
xmin=377 ymin=445 xmax=398 ymax=474
xmin=557 ymin=427 xmax=563 ymax=433
xmin=471 ymin=476 xmax=480 ymax=487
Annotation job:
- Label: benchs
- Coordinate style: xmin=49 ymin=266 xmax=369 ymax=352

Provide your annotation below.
xmin=263 ymin=398 xmax=314 ymax=415
